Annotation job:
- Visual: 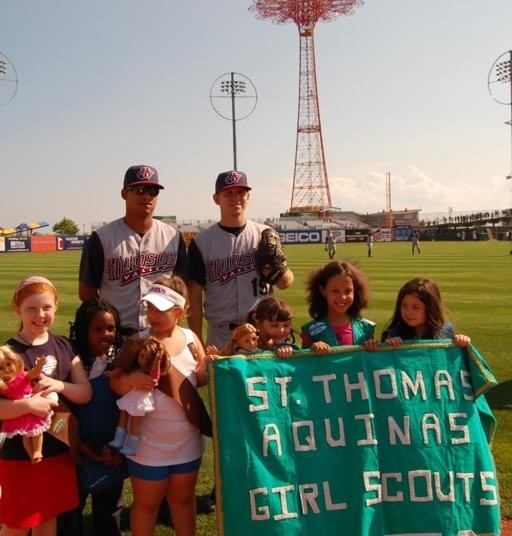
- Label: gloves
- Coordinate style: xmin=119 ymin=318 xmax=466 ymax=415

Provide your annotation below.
xmin=255 ymin=249 xmax=288 ymax=285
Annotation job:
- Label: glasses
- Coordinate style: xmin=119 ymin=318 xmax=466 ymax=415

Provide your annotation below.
xmin=126 ymin=185 xmax=159 ymax=196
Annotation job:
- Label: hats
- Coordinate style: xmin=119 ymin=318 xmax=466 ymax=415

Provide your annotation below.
xmin=327 ymin=230 xmax=331 ymax=233
xmin=216 ymin=171 xmax=252 ymax=193
xmin=124 ymin=165 xmax=164 ymax=190
xmin=137 ymin=284 xmax=186 ymax=312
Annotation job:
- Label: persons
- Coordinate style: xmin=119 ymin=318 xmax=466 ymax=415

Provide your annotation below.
xmin=0 ymin=345 xmax=49 ymax=464
xmin=244 ymin=296 xmax=300 ymax=360
xmin=381 ymin=276 xmax=473 ymax=348
xmin=79 ymin=165 xmax=186 ymax=335
xmin=58 ymin=297 xmax=126 ymax=536
xmin=410 ymin=229 xmax=421 ymax=256
xmin=227 ymin=325 xmax=262 ymax=358
xmin=325 ymin=229 xmax=338 ymax=260
xmin=298 ymin=261 xmax=381 ymax=352
xmin=112 ymin=276 xmax=209 ymax=535
xmin=111 ymin=335 xmax=166 ymax=455
xmin=182 ymin=169 xmax=294 ymax=354
xmin=1 ymin=275 xmax=92 ymax=535
xmin=367 ymin=232 xmax=374 ymax=257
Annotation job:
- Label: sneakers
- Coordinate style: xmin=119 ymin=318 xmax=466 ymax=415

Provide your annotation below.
xmin=210 ymin=491 xmax=216 ymax=508
